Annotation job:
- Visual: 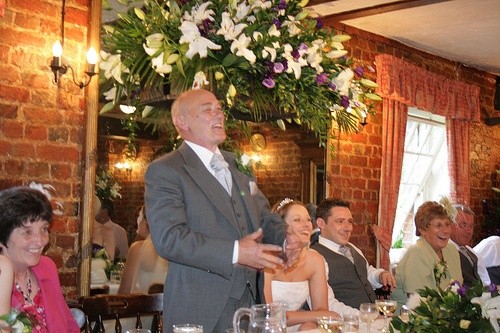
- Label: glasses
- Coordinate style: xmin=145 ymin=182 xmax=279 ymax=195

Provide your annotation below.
xmin=455 ymin=223 xmax=476 ymax=232
xmin=427 ymin=221 xmax=455 ymax=231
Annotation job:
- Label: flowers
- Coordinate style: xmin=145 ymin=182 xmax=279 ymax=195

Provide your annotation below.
xmin=439 ymin=196 xmax=458 ymax=221
xmin=94 ymin=248 xmax=126 ymax=272
xmin=95 ymin=169 xmax=122 ymax=201
xmin=433 ymin=260 xmax=449 ymax=286
xmin=389 ymin=280 xmax=500 ymax=333
xmin=95 ymin=0 xmax=382 ymax=159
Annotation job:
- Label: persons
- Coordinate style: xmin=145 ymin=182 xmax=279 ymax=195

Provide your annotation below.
xmin=390 ymin=201 xmax=463 ymax=315
xmin=446 ymin=204 xmax=483 ymax=296
xmin=264 ymin=197 xmax=342 ymax=333
xmin=473 ymin=236 xmax=500 ymax=287
xmin=92 ymin=195 xmax=128 ymax=266
xmin=0 ymin=187 xmax=81 ymax=333
xmin=144 ymin=88 xmax=304 ymax=333
xmin=310 ymin=198 xmax=400 ymax=333
xmin=117 ymin=205 xmax=169 ymax=293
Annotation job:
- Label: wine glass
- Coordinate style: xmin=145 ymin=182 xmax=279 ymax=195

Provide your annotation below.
xmin=359 ymin=302 xmax=379 ymax=333
xmin=374 ymin=299 xmax=397 ymax=333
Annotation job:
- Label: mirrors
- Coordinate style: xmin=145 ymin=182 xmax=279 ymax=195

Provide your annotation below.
xmin=89 ymin=1 xmax=327 ymax=333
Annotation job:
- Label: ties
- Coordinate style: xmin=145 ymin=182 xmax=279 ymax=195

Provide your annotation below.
xmin=459 ymin=245 xmax=481 ymax=281
xmin=210 ymin=153 xmax=234 ymax=198
xmin=338 ymin=244 xmax=355 ymax=266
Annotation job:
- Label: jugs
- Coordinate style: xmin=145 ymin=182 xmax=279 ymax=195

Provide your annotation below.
xmin=233 ymin=299 xmax=289 ymax=333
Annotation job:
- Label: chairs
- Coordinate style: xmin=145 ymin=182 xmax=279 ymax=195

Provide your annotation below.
xmin=79 ymin=293 xmax=163 ymax=333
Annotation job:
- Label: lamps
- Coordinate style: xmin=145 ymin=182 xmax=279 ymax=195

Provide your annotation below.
xmin=48 ymin=41 xmax=97 ymax=90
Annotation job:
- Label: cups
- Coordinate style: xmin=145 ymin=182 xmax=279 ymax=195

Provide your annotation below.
xmin=113 ymin=271 xmax=121 ymax=284
xmin=126 ymin=328 xmax=150 ymax=333
xmin=401 ymin=305 xmax=409 ymax=323
xmin=172 ymin=323 xmax=204 ymax=332
xmin=316 ymin=313 xmax=359 ymax=333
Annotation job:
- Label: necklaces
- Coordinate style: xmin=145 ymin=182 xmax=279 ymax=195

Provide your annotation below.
xmin=16 ymin=276 xmax=34 ymax=305
